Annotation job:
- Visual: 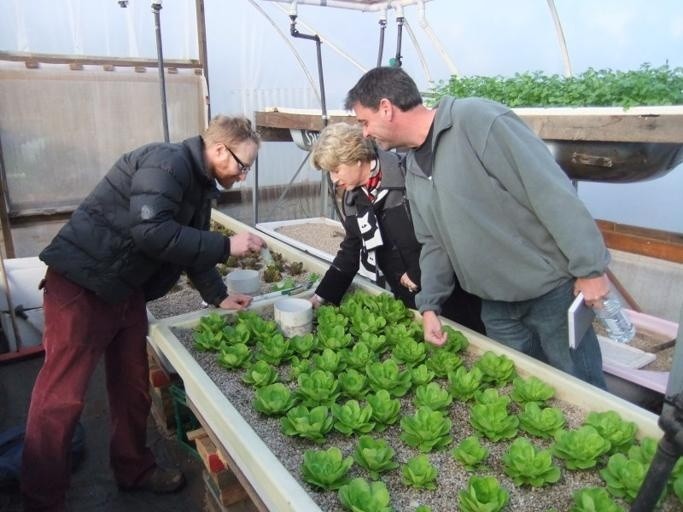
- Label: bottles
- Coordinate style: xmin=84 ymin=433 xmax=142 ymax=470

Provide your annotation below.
xmin=592 ymin=294 xmax=637 ymax=346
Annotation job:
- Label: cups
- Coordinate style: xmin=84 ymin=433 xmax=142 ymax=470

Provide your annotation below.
xmin=273 ymin=297 xmax=313 ymax=339
xmin=226 ymin=269 xmax=259 ymax=299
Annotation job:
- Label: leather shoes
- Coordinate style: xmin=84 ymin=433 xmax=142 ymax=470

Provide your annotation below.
xmin=139 ymin=464 xmax=186 ymax=496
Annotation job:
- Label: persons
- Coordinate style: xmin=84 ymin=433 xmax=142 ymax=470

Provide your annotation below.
xmin=308 ymin=122 xmax=486 ymax=334
xmin=19 ymin=115 xmax=268 ymax=512
xmin=344 ymin=67 xmax=613 ymax=393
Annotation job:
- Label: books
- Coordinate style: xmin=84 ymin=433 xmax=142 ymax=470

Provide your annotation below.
xmin=567 ymin=293 xmax=596 ymax=349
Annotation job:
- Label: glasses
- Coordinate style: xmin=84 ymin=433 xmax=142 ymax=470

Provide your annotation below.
xmin=225 ymin=145 xmax=252 ymax=175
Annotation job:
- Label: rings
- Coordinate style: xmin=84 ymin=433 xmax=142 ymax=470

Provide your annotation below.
xmin=408 ymin=288 xmax=413 ymax=293
